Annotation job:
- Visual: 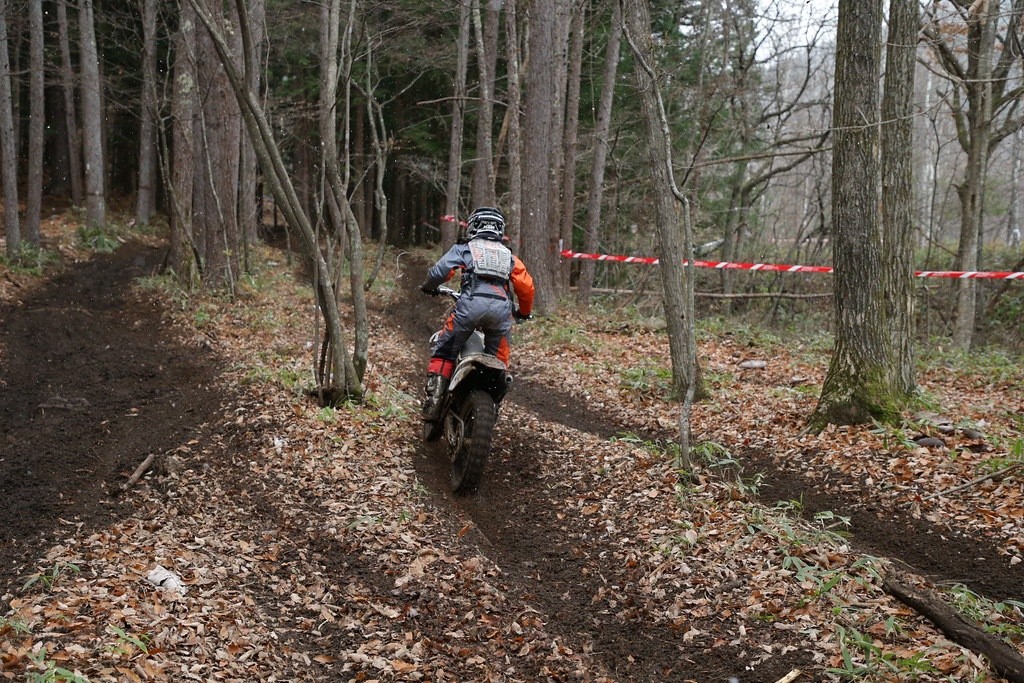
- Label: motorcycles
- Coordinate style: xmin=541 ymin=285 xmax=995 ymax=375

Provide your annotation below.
xmin=415 ymin=279 xmax=514 ymax=502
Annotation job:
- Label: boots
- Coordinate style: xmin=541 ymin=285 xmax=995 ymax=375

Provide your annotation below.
xmin=421 ymin=372 xmax=449 ymax=420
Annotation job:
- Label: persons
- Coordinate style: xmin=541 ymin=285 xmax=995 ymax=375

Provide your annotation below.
xmin=420 ymin=207 xmax=534 ymax=419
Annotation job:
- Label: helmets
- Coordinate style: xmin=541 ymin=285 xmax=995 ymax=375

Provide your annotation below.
xmin=466 ymin=207 xmax=505 ymax=244
